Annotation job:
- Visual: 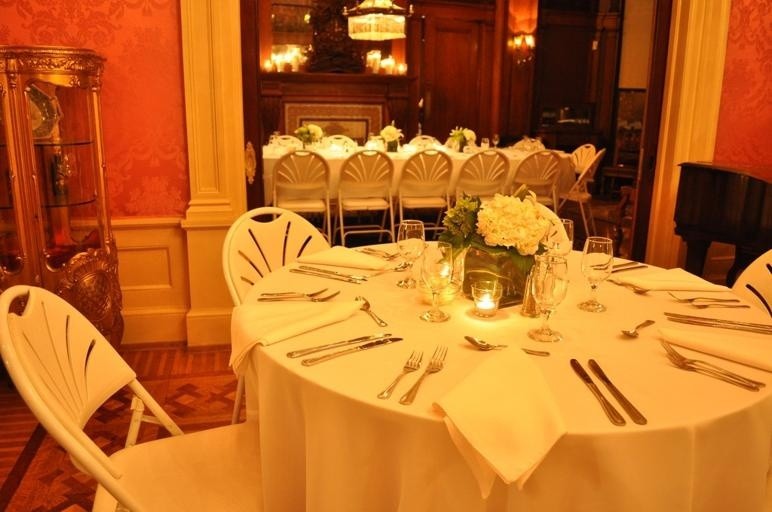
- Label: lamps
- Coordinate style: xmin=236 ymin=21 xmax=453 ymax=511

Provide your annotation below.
xmin=342 ymin=0 xmax=415 ymax=40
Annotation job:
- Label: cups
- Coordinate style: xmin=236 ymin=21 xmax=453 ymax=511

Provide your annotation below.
xmin=469 ymin=278 xmax=501 ymax=319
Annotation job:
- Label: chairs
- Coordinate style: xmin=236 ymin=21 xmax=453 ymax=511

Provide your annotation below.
xmin=221 ymin=207 xmax=330 ymax=424
xmin=730 ymin=249 xmax=772 ymax=318
xmin=0 ymin=285 xmax=263 ymax=512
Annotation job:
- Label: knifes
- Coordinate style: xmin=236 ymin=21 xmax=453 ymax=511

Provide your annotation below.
xmin=289 ymin=264 xmax=368 ymax=285
xmin=302 ymin=338 xmax=404 ymax=367
xmin=587 ymin=358 xmax=652 ymax=427
xmin=662 ymin=310 xmax=772 ymax=338
xmin=286 ymin=333 xmax=393 ymax=358
xmin=566 ymin=353 xmax=625 ymax=432
xmin=587 ymin=259 xmax=648 ymax=275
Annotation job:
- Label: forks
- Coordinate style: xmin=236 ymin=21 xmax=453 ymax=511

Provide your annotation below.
xmin=659 ymin=339 xmax=767 ymax=393
xmin=397 ymin=343 xmax=450 ymax=407
xmin=376 ymin=349 xmax=423 ymax=407
xmin=354 ymin=245 xmax=400 ymax=262
xmin=256 ymin=285 xmax=342 ymax=304
xmin=666 ymin=290 xmax=752 ymax=309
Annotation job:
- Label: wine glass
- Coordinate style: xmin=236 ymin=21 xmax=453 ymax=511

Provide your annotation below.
xmin=526 ymin=255 xmax=565 ymax=345
xmin=395 ymin=215 xmax=465 ymax=323
xmin=550 ymin=216 xmax=575 ymax=294
xmin=479 ymin=131 xmax=546 ymax=156
xmin=577 ymin=236 xmax=613 ymax=315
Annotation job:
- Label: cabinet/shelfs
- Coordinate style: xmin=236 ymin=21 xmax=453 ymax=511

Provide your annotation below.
xmin=0 ymin=45 xmax=124 ymax=351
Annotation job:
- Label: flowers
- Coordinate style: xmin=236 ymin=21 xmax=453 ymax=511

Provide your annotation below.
xmin=436 ymin=190 xmax=572 ymax=272
xmin=294 ymin=123 xmax=323 ymax=142
xmin=380 ymin=126 xmax=404 ymax=140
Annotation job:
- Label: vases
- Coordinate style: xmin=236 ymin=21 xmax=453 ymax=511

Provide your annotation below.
xmin=463 ymin=247 xmax=536 ymax=309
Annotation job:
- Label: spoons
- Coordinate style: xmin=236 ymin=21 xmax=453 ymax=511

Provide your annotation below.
xmin=620 ymin=316 xmax=656 ymax=339
xmin=354 ymin=294 xmax=388 ymax=328
xmin=605 ymin=277 xmax=651 ymax=298
xmin=462 ymin=333 xmax=553 ymax=357
xmin=363 ymin=260 xmax=414 ymax=278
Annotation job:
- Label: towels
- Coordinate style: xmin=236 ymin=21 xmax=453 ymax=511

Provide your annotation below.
xmin=608 ymin=268 xmax=732 ymax=291
xmin=228 ymin=300 xmax=366 ymax=374
xmin=656 ymin=327 xmax=772 ymax=372
xmin=434 ymin=345 xmax=567 ymax=501
xmin=297 ymin=245 xmax=399 ymax=271
